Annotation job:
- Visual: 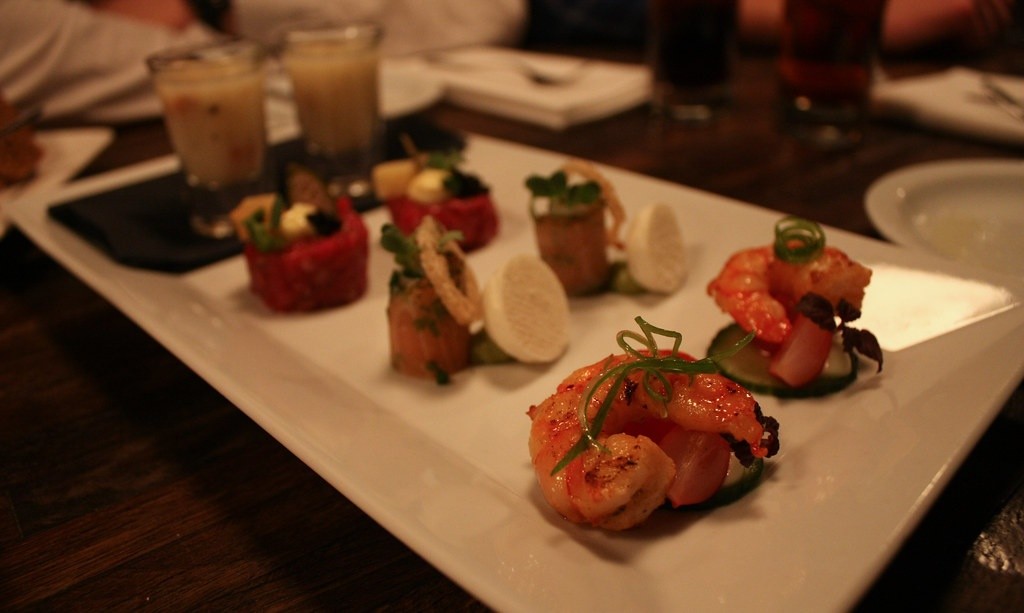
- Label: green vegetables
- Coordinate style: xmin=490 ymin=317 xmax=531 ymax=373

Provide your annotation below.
xmin=235 ymin=147 xmax=826 ymax=479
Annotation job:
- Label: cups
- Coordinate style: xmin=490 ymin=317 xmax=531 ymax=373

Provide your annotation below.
xmin=778 ymin=0 xmax=884 ymax=155
xmin=144 ymin=37 xmax=274 ymax=236
xmin=279 ymin=18 xmax=386 ymax=171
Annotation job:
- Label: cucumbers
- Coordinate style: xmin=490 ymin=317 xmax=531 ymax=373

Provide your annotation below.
xmin=710 ymin=322 xmax=862 ymax=397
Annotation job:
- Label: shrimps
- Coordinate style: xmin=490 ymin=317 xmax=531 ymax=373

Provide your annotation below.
xmin=528 ymin=348 xmax=773 ymax=533
xmin=707 ymin=240 xmax=872 ymax=346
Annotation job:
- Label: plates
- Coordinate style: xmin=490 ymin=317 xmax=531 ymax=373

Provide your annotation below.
xmin=1 ymin=127 xmax=112 ymax=234
xmin=443 ymin=45 xmax=655 ymax=130
xmin=871 ymin=67 xmax=1023 ymax=141
xmin=0 ymin=125 xmax=1023 ymax=613
xmin=863 ymin=159 xmax=1024 ymax=281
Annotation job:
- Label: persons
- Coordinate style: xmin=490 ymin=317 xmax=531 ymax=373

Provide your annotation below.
xmin=0 ymin=0 xmax=232 ymax=126
xmin=781 ymin=0 xmax=1016 ymax=66
xmin=491 ymin=1 xmax=740 ymax=89
